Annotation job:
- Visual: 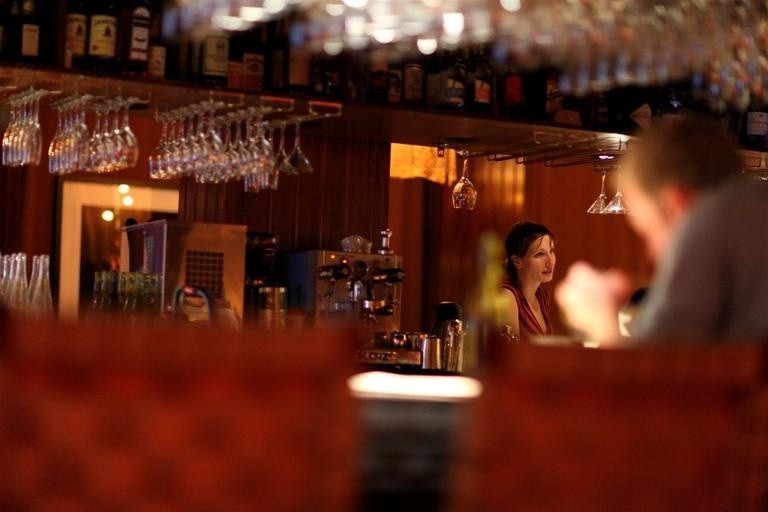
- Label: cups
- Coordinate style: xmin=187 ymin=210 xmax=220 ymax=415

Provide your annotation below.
xmin=420 ymin=338 xmax=443 ymax=370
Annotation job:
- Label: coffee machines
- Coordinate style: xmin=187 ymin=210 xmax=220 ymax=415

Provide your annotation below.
xmin=243 ymin=231 xmax=290 ymax=334
xmin=303 ymin=250 xmax=420 ymax=364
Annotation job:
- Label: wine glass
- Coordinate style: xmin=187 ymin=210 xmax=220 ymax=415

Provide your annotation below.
xmin=448 ymin=149 xmax=479 ymax=210
xmin=585 ymin=168 xmax=633 ymax=216
xmin=1 ymin=86 xmax=315 ymax=200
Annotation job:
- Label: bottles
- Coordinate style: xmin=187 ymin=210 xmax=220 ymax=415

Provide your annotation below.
xmin=0 ymin=0 xmax=766 ymax=151
xmin=86 ymin=268 xmax=160 ymax=323
xmin=0 ymin=253 xmax=56 ymax=318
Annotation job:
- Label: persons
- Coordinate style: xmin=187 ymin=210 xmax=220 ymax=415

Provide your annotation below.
xmin=496 ymin=220 xmax=556 ymax=342
xmin=554 ymin=114 xmax=768 ymax=350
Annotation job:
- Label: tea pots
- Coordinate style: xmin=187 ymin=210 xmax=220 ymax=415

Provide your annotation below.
xmin=434 ymin=300 xmax=463 ymax=373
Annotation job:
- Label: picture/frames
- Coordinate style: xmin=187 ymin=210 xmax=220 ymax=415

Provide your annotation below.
xmin=49 ymin=169 xmax=179 ymax=325
